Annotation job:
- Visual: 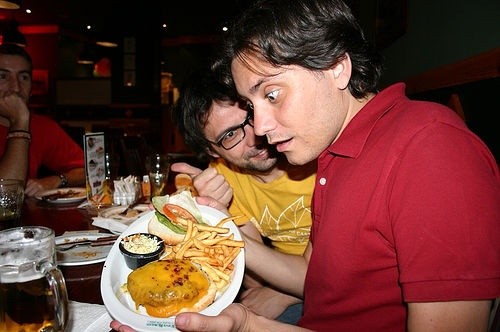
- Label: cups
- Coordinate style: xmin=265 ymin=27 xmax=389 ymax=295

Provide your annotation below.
xmin=0 ymin=226 xmax=70 ymax=332
xmin=85 ymin=153 xmax=112 ymax=208
xmin=119 ymin=233 xmax=165 ymax=270
xmin=0 ymin=178 xmax=25 ymax=225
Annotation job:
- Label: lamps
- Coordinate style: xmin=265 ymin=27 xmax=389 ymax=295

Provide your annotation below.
xmin=96 ymin=23 xmax=119 ymax=48
xmin=78 ymin=45 xmax=94 ymax=64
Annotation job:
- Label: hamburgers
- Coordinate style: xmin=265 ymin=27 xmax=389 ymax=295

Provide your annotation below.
xmin=127 ymin=190 xmax=216 ymax=317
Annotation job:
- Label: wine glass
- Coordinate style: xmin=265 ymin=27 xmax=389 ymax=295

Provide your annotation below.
xmin=149 ymin=153 xmax=171 ymax=196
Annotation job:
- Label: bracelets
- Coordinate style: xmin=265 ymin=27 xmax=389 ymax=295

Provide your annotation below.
xmin=6 ymin=130 xmax=31 ymax=141
xmin=57 ymin=174 xmax=68 ymax=188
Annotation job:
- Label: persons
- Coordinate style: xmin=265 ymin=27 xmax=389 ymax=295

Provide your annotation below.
xmin=109 ymin=0 xmax=500 ymax=332
xmin=0 ymin=43 xmax=86 ymax=199
xmin=171 ymin=70 xmax=318 ymax=326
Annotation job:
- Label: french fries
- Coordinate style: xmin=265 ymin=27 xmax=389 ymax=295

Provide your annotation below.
xmin=160 ymin=214 xmax=246 ymax=291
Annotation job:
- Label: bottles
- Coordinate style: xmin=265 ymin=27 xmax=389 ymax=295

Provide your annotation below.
xmin=142 ymin=175 xmax=151 ymax=196
xmin=134 ymin=176 xmax=142 ymax=201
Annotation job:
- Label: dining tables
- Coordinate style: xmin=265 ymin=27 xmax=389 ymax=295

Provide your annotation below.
xmin=0 ymin=180 xmax=211 ymax=332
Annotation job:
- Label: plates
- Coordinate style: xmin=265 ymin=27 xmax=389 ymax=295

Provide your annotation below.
xmin=53 ymin=232 xmax=117 ymax=267
xmin=34 ymin=187 xmax=87 ymax=203
xmin=99 ymin=204 xmax=245 ymax=332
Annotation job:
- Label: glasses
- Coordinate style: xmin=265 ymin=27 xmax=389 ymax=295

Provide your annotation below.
xmin=202 ymin=114 xmax=254 ymax=150
